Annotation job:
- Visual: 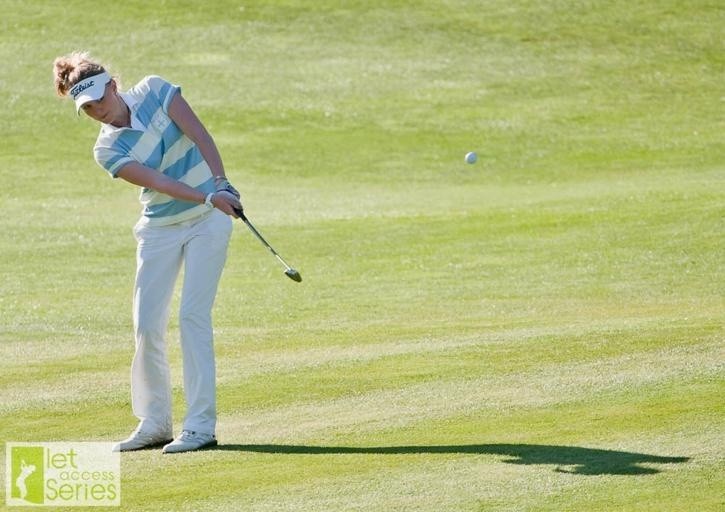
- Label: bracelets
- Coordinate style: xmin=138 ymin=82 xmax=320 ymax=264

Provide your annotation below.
xmin=214 ymin=175 xmax=228 ymax=183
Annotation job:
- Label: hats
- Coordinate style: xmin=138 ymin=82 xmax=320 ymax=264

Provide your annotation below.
xmin=68 ymin=71 xmax=112 ymax=117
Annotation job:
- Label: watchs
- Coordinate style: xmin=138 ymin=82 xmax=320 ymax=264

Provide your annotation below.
xmin=204 ymin=193 xmax=214 ymax=211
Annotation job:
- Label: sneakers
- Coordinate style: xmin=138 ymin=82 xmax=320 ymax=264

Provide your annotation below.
xmin=113 ymin=426 xmax=174 ymax=452
xmin=163 ymin=427 xmax=218 ymax=453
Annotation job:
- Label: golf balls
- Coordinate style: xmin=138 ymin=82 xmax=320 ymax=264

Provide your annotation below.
xmin=465 ymin=152 xmax=477 ymax=164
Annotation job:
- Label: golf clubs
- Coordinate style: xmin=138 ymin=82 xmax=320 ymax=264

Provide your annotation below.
xmin=231 ymin=205 xmax=301 ymax=282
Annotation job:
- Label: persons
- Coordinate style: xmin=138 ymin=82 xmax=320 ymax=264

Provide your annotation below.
xmin=51 ymin=48 xmax=246 ymax=455
